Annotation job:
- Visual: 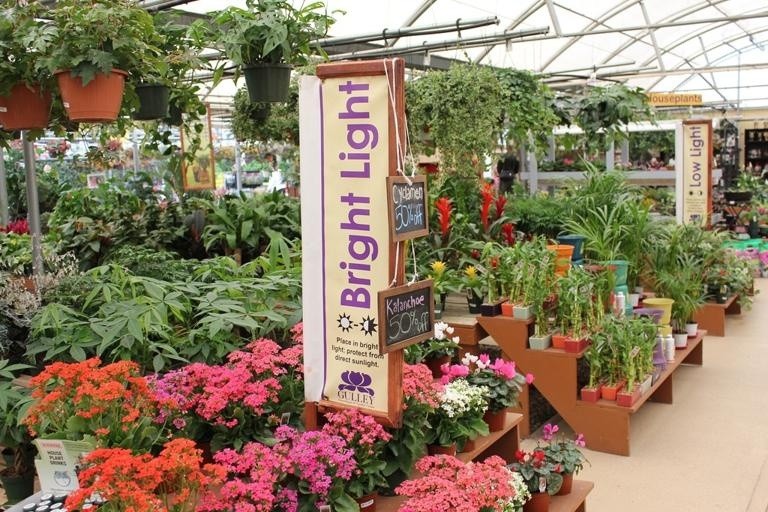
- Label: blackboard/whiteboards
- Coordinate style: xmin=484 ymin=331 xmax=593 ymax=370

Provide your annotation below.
xmin=378 ymin=278 xmax=434 ymax=354
xmin=386 ymin=175 xmax=430 ymax=243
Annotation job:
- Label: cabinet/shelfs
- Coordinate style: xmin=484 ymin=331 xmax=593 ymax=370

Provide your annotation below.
xmin=743 ymin=128 xmax=768 ymax=176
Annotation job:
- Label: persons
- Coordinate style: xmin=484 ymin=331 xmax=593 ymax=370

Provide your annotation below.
xmin=497 ymin=148 xmax=520 ymax=194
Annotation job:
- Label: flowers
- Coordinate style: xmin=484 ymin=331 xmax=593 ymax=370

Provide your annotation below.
xmin=20 ymin=323 xmax=592 ymax=512
xmin=424 ymin=186 xmax=521 ymax=304
xmin=735 ymin=247 xmax=768 ymax=266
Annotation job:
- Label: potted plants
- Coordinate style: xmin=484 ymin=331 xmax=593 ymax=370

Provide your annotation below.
xmin=499 ymin=191 xmax=722 ymax=408
xmin=0 ymin=0 xmax=348 ymax=150
xmin=574 ymin=81 xmax=660 ymax=157
